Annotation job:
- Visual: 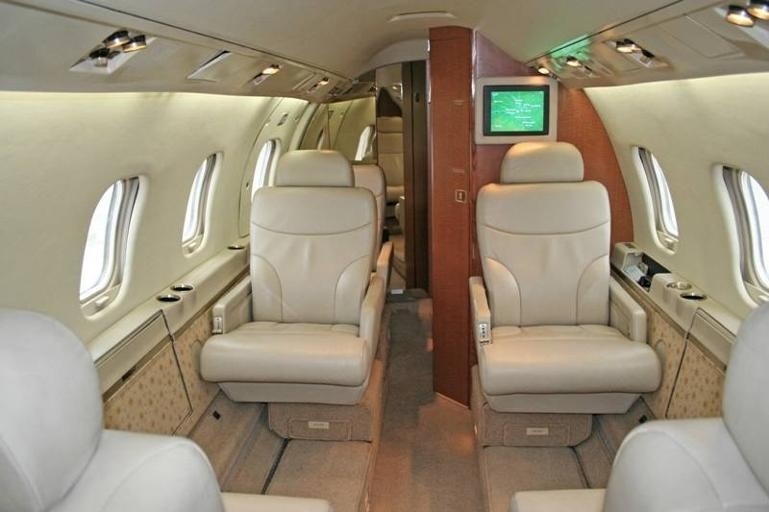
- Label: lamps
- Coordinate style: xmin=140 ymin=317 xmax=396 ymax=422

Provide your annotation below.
xmin=616 ymin=41 xmax=655 ymax=67
xmin=89 ymin=28 xmax=148 ymax=70
xmin=723 ymin=1 xmax=769 ymax=29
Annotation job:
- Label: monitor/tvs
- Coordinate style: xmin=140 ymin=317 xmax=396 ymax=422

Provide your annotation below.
xmin=475 ymin=76 xmax=558 ymax=144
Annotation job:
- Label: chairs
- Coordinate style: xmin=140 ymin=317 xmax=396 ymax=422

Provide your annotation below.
xmin=0 ymin=299 xmax=331 ymax=510
xmin=511 ymin=298 xmax=769 ymax=510
xmin=192 ymin=148 xmax=396 ymax=410
xmin=459 ymin=138 xmax=666 ymax=420
